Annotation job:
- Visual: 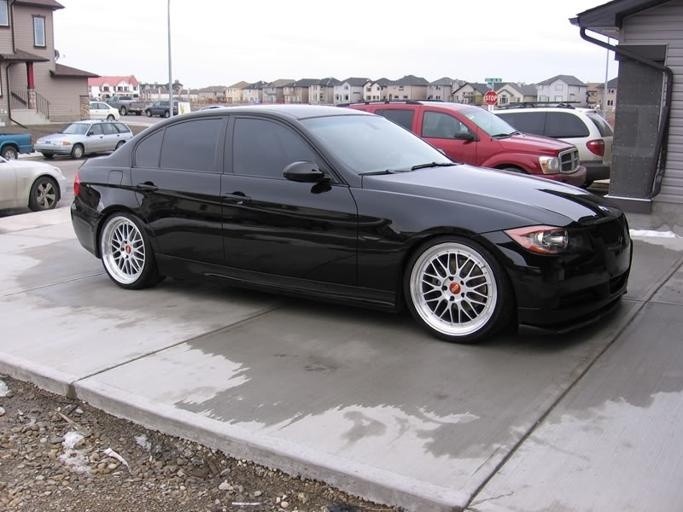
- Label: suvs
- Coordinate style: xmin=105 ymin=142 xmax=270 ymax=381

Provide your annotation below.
xmin=485 ymin=97 xmax=616 ymax=191
xmin=332 ymin=97 xmax=594 ymax=195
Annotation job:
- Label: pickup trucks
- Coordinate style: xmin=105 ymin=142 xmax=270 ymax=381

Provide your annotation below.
xmin=104 ymin=94 xmax=144 ymax=116
xmin=0 ymin=132 xmax=36 ymax=161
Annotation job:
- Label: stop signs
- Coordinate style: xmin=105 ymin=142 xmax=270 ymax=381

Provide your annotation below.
xmin=482 ymin=90 xmax=498 ymax=106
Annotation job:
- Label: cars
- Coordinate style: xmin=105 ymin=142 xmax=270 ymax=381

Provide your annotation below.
xmin=89 ymin=100 xmax=120 ymax=120
xmin=68 ymin=100 xmax=633 ymax=349
xmin=31 ymin=117 xmax=135 ymax=162
xmin=0 ymin=154 xmax=68 ymax=212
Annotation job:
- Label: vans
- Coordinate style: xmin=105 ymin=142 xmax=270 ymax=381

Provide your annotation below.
xmin=142 ymin=98 xmax=179 ymax=119
